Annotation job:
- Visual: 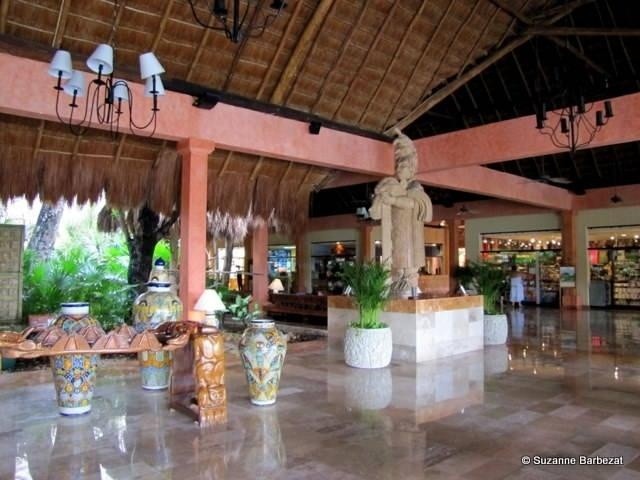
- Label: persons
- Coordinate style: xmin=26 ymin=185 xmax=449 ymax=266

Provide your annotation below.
xmin=509 ymin=306 xmax=526 ymax=344
xmin=508 ymin=265 xmax=526 ymax=306
xmin=236 ymin=264 xmax=245 ymax=292
xmin=369 ymin=128 xmax=434 ymax=291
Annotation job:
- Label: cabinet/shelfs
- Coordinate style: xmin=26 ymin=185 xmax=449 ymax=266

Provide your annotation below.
xmin=485 ymin=248 xmax=560 ymax=307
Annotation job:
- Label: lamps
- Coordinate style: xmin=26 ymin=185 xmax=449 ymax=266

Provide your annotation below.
xmin=48 ymin=39 xmax=166 ymax=145
xmin=188 ymin=1 xmax=288 ymax=45
xmin=531 ymin=78 xmax=614 ymax=159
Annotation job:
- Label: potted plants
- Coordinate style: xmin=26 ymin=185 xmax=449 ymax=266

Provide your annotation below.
xmin=334 ymin=252 xmax=409 ymax=369
xmin=470 ymin=258 xmax=512 ymax=347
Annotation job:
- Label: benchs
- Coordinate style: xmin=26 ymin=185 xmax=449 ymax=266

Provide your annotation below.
xmin=260 ymin=292 xmax=328 ymax=325
